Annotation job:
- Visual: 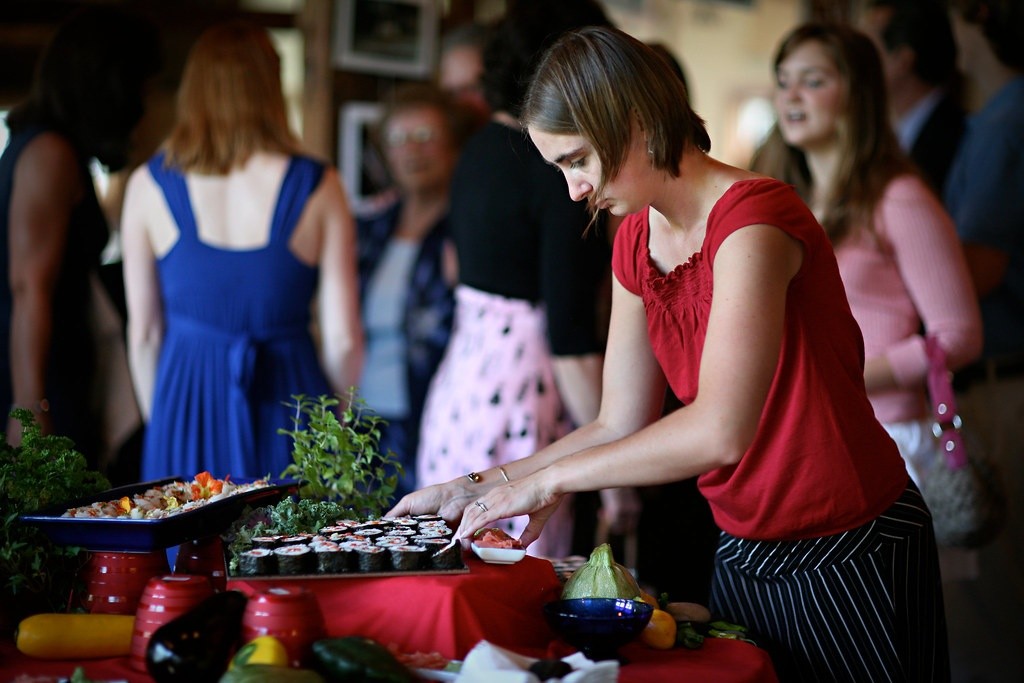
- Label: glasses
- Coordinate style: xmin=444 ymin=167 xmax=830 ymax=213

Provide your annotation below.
xmin=383 ymin=126 xmax=433 ymax=146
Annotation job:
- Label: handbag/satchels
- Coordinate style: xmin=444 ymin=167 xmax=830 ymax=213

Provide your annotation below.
xmin=912 ymin=336 xmax=1008 ymax=547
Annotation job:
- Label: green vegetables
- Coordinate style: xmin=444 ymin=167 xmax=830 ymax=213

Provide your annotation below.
xmin=221 ymin=495 xmax=382 ymax=572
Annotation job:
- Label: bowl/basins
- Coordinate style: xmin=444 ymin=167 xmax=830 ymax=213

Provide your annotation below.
xmin=547 ymin=596 xmax=653 ymax=664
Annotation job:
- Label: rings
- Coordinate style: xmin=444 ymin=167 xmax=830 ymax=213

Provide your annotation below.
xmin=474 ymin=500 xmax=488 ymax=512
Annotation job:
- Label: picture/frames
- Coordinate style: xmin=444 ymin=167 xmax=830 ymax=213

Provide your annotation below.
xmin=328 ymin=0 xmax=442 ymax=220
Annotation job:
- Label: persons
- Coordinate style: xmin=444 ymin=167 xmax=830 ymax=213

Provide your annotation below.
xmin=98 ymin=16 xmax=366 ymax=572
xmin=1 ymin=0 xmax=1023 ymax=683
xmin=381 ymin=26 xmax=949 ymax=683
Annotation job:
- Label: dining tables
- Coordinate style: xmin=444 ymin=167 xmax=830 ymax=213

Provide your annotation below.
xmin=0 ymin=629 xmax=778 ymax=683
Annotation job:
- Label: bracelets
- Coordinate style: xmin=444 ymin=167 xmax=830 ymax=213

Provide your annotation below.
xmin=466 ymin=465 xmax=511 ymax=483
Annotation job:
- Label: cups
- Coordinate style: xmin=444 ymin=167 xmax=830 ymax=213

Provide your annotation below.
xmin=243 ymin=586 xmax=319 ymax=668
xmin=126 ymin=575 xmax=208 ymax=673
xmin=176 ymin=538 xmax=226 ymax=601
xmin=88 ymin=550 xmax=168 ymax=622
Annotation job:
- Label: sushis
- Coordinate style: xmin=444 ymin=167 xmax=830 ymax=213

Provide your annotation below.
xmin=239 ymin=513 xmax=464 ymax=576
xmin=60 ymin=471 xmax=277 ymax=520
